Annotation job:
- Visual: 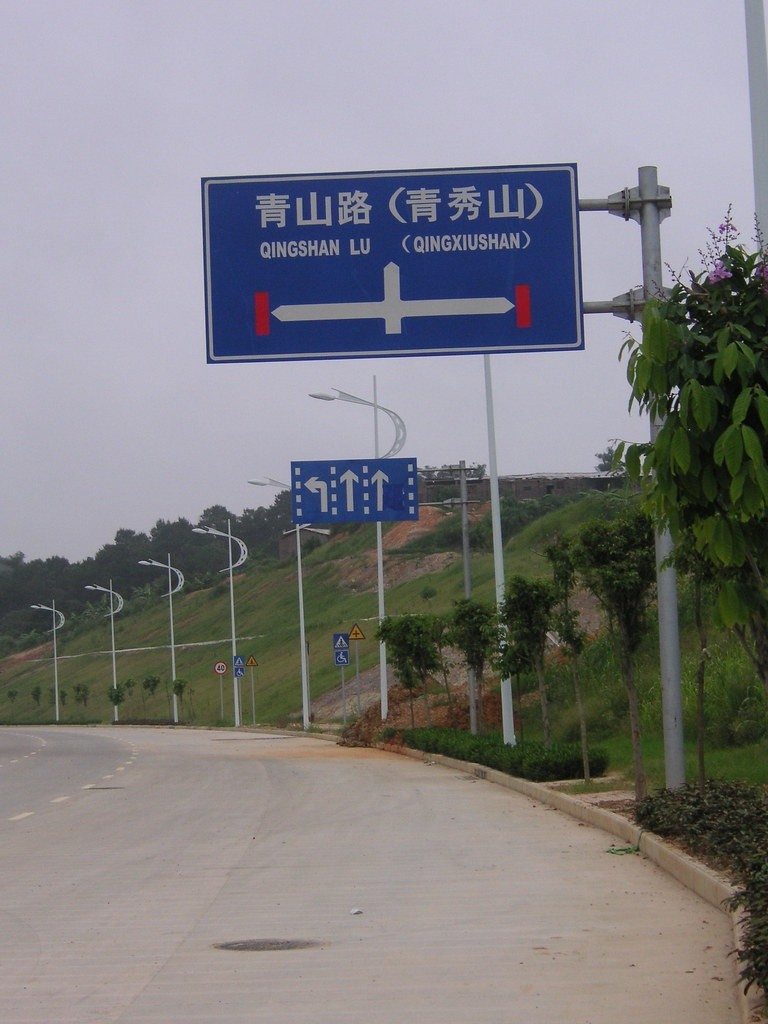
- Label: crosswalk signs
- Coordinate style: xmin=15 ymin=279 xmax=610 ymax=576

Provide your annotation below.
xmin=234 ymin=655 xmax=246 ymax=666
xmin=334 ymin=633 xmax=350 ymax=648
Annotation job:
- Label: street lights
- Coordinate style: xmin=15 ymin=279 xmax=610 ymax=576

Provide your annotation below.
xmin=307 ymin=386 xmax=408 ymax=717
xmin=248 ymin=476 xmax=332 ymax=732
xmin=84 ymin=579 xmax=124 ymax=722
xmin=190 ymin=518 xmax=250 ymax=726
xmin=138 ymin=552 xmax=186 ymax=725
xmin=30 ymin=599 xmax=66 ymax=721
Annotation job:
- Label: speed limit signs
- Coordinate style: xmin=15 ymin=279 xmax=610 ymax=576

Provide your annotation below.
xmin=215 ymin=662 xmax=226 ymax=676
